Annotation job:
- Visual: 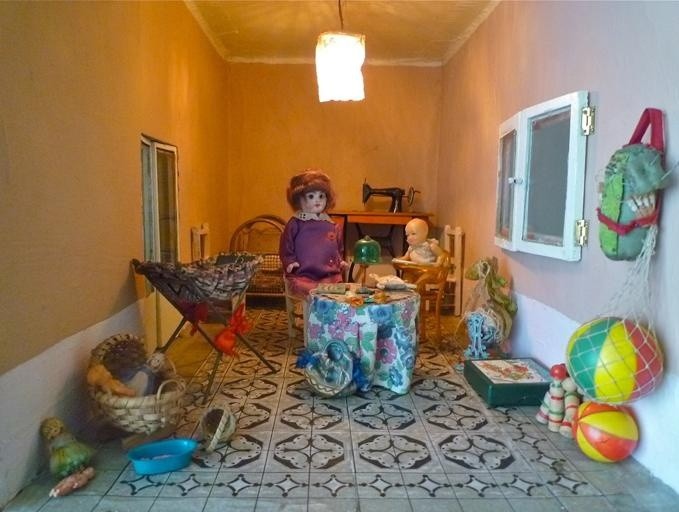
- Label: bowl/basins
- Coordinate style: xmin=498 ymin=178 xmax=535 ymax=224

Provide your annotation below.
xmin=126 ymin=438 xmax=197 ymax=475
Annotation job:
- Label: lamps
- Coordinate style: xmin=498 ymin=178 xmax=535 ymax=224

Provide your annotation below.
xmin=352 ymin=235 xmax=381 ymax=296
xmin=314 ymin=0 xmax=366 ymax=103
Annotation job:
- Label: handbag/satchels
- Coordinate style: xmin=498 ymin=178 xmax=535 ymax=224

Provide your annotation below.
xmin=596 ymin=143 xmax=672 ymax=260
xmin=304 ymin=340 xmax=357 ymax=399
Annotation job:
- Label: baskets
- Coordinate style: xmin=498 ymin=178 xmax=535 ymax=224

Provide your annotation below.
xmin=88 ymin=333 xmax=186 ymax=436
xmin=200 ymin=407 xmax=236 ymax=454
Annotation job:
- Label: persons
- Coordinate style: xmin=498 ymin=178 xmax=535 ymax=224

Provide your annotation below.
xmin=39 ymin=417 xmax=96 ymax=471
xmin=47 ymin=467 xmax=96 ymax=500
xmin=83 ymin=350 xmax=170 ymax=398
xmin=395 ymin=217 xmax=447 ymax=282
xmin=280 ymin=170 xmax=352 ymax=296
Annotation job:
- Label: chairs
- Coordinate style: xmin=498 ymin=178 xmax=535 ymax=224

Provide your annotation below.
xmin=389 ymin=250 xmax=451 ymax=344
xmin=280 ymin=273 xmax=307 ymax=339
xmin=425 ymin=224 xmax=463 ymax=317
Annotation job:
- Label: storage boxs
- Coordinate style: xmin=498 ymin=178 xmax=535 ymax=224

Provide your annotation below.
xmin=462 ymin=356 xmax=555 ymax=410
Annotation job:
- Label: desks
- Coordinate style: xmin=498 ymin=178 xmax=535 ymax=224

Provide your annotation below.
xmin=328 ymin=210 xmax=437 ymax=283
xmin=302 ymin=283 xmax=422 ymax=400
